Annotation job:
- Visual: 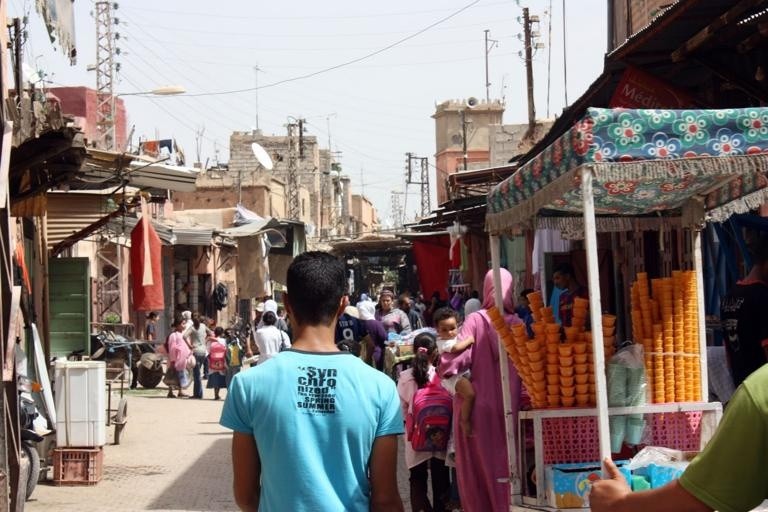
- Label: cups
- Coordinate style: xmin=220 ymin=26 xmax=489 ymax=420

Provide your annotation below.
xmin=607 ymin=363 xmax=646 ymax=454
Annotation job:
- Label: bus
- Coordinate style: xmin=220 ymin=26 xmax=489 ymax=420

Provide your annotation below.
xmin=331 ymin=230 xmax=420 ymax=304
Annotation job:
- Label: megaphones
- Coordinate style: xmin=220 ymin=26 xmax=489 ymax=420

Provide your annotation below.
xmin=466 ymin=96 xmax=479 ymax=109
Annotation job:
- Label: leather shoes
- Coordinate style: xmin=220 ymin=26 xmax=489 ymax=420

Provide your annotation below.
xmin=188 ymin=395 xmax=199 ymax=398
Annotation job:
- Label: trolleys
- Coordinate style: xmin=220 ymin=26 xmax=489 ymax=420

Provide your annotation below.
xmin=51 ymin=366 xmax=129 ymax=447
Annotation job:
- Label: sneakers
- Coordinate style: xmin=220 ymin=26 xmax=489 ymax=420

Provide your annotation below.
xmin=178 ymin=394 xmax=187 ymax=398
xmin=167 ymin=394 xmax=175 ymax=398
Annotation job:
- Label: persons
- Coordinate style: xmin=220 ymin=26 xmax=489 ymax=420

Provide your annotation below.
xmin=396 ymin=330 xmax=454 ymax=511
xmin=147 ymin=311 xmax=160 ymax=347
xmin=432 ymin=306 xmax=476 ymax=438
xmin=588 ymin=361 xmax=766 ymax=511
xmin=166 ymin=263 xmax=589 ymax=401
xmin=436 ymin=266 xmax=528 ymax=512
xmin=215 ymin=251 xmax=405 ymax=512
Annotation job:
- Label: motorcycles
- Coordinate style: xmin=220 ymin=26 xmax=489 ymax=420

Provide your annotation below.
xmin=18 ymin=374 xmax=46 ymax=502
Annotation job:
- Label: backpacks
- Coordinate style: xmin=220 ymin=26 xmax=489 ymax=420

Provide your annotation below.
xmin=209 ymin=341 xmax=227 ymax=370
xmin=405 ymin=373 xmax=452 ymax=453
xmin=225 ymin=339 xmax=242 ymax=368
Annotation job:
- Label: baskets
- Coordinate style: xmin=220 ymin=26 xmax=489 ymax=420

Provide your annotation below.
xmin=636 ymin=401 xmax=700 ymax=453
xmin=534 ymin=406 xmax=599 ymax=463
